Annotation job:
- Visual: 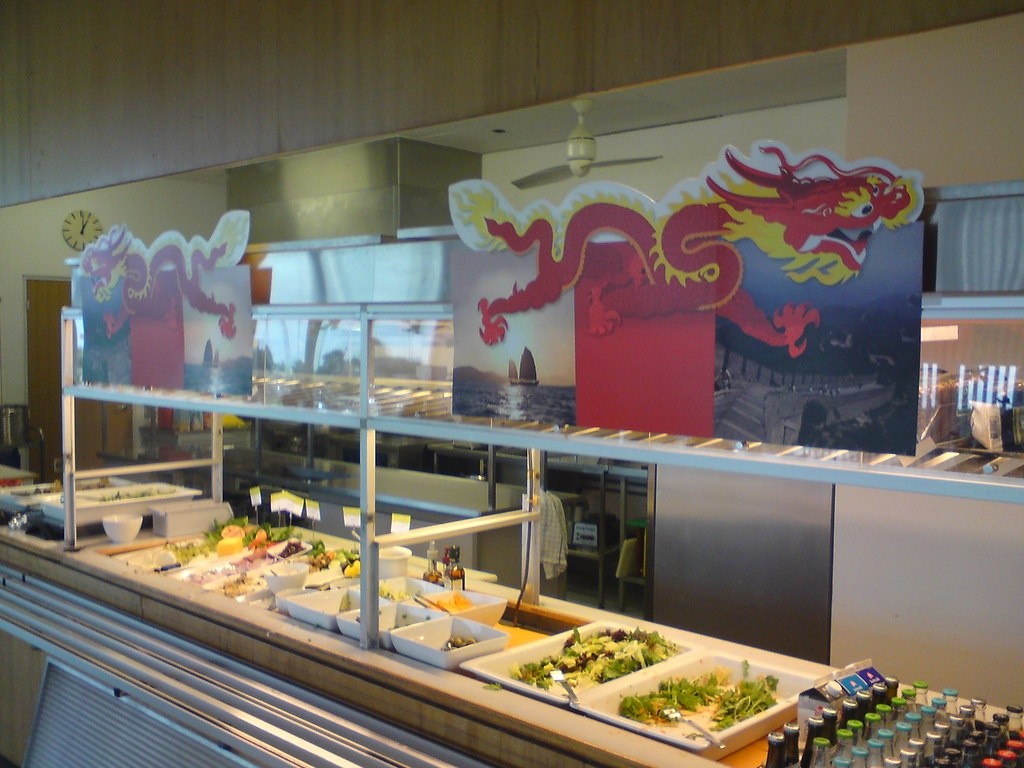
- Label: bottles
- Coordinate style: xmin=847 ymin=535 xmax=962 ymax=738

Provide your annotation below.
xmin=764 ymin=676 xmax=1024 ymax=768
xmin=443 ymin=544 xmax=464 ymax=590
xmin=423 ymin=540 xmax=442 ymax=586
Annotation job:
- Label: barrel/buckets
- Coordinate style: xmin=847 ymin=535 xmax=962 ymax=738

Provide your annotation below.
xmin=0 ymin=405 xmax=30 ymax=450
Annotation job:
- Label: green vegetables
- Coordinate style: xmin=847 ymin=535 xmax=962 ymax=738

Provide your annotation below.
xmin=206 ymin=517 xmax=359 ymax=564
xmin=620 ymin=660 xmax=779 ymax=732
xmin=518 ymin=625 xmax=679 ymax=690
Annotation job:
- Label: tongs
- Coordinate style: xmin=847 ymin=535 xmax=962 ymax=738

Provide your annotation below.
xmin=548 ymin=670 xmax=580 ymax=705
xmin=414 ymin=593 xmax=451 ymax=616
xmin=658 ymin=707 xmax=726 ymax=749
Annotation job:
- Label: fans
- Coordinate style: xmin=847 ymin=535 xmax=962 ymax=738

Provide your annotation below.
xmin=511 ymin=98 xmax=664 ymax=190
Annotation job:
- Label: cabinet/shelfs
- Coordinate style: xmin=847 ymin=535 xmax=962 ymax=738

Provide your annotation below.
xmin=1 ymin=293 xmax=1024 ymax=768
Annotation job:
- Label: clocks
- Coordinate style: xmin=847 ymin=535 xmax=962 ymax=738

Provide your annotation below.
xmin=62 ymin=210 xmax=102 ymax=251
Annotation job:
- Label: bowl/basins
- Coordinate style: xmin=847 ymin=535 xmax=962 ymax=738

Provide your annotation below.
xmin=287 ymin=586 xmax=390 ymax=631
xmin=337 ymin=601 xmax=448 ymax=651
xmin=390 ymin=615 xmax=509 ymax=675
xmin=264 ymin=563 xmax=310 ymax=595
xmin=102 ymin=513 xmax=144 ymax=543
xmin=349 ymin=576 xmax=445 ymax=603
xmin=399 ymin=588 xmax=507 ymax=628
xmin=276 ymin=588 xmax=320 ymax=615
xmin=379 ymin=546 xmax=412 ymax=580
